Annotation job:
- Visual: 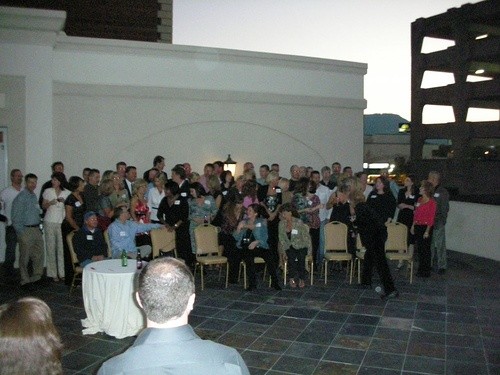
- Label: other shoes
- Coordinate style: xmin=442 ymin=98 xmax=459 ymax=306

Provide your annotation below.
xmin=246 ymin=285 xmax=256 ymax=290
xmin=272 ymin=282 xmax=281 ymax=290
xmin=290 ymin=279 xmax=296 ymax=288
xmin=414 ymin=263 xmax=433 ymax=276
xmin=297 ymin=278 xmax=304 ymax=289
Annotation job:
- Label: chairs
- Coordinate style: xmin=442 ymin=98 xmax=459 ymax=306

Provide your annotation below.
xmin=237 ymin=257 xmax=272 ymax=289
xmin=386 ymin=222 xmax=413 ymax=285
xmin=281 ymin=224 xmax=313 ymax=286
xmin=194 ymin=223 xmax=229 ymax=291
xmin=66 ymin=230 xmax=83 ymax=296
xmin=104 ymin=230 xmax=112 ymax=258
xmin=353 ymin=233 xmax=366 ymax=284
xmin=150 ymin=229 xmax=185 ymax=264
xmin=320 ymin=221 xmax=353 ymax=285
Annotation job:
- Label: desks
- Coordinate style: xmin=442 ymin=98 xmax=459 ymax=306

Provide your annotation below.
xmin=81 ymin=259 xmax=150 ymax=339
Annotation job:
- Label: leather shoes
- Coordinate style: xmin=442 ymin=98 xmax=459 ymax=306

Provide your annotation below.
xmin=380 ymin=289 xmax=400 ymax=299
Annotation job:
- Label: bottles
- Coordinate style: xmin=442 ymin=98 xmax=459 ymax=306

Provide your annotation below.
xmin=121 ymin=249 xmax=128 ymax=266
xmin=137 ymin=249 xmax=142 ymax=269
xmin=204 ymin=216 xmax=208 ymax=227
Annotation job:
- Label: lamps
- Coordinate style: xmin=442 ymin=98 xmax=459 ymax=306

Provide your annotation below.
xmin=223 ymin=154 xmax=237 ymax=176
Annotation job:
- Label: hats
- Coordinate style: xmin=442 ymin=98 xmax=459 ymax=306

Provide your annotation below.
xmin=84 ymin=211 xmax=95 ymax=223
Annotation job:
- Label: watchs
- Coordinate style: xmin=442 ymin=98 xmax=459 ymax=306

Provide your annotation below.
xmin=174 ymin=223 xmax=178 ymax=227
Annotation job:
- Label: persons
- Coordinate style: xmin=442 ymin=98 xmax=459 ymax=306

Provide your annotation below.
xmin=410 ymin=180 xmax=436 ymax=279
xmin=233 ymin=204 xmax=277 ymax=282
xmin=0 ymin=154 xmax=372 ymax=281
xmin=366 ymin=176 xmax=397 ymax=228
xmin=72 ymin=210 xmax=109 ymax=267
xmin=278 ymin=203 xmax=312 ymax=287
xmin=394 ymin=176 xmax=420 ymax=272
xmin=0 ymin=296 xmax=64 ymax=375
xmin=96 ymin=257 xmax=250 ymax=375
xmin=427 ymin=170 xmax=449 ymax=273
xmin=348 ymin=189 xmax=400 ymax=298
xmin=12 ymin=172 xmax=54 ymax=294
xmin=108 ymin=206 xmax=165 ymax=259
